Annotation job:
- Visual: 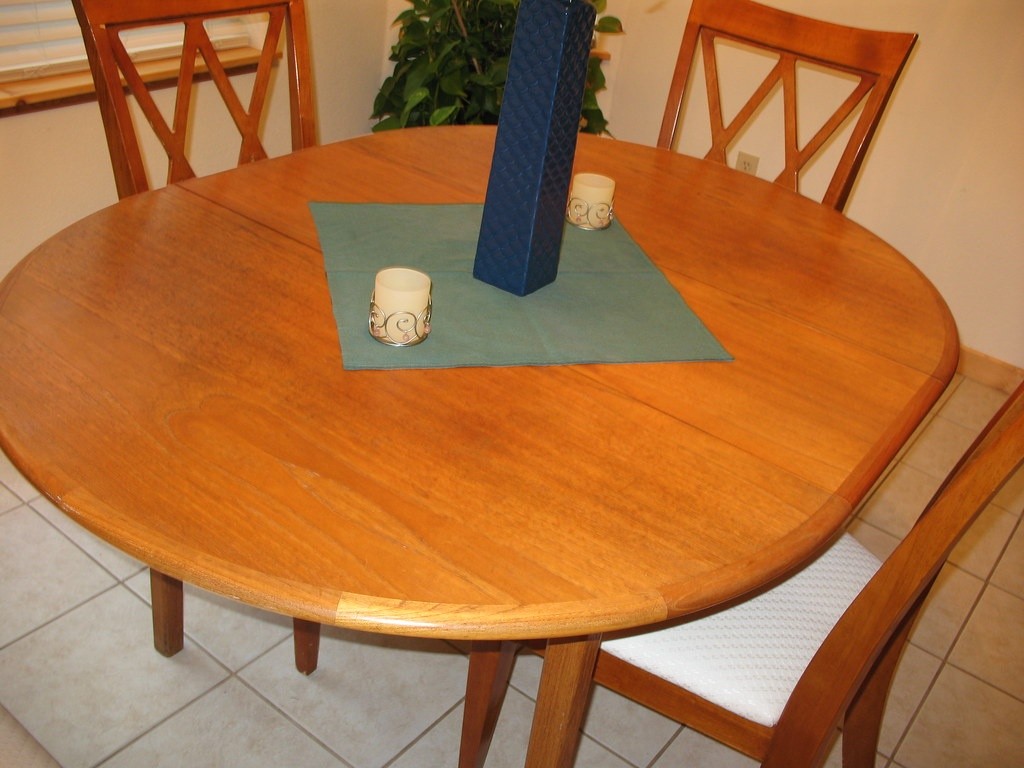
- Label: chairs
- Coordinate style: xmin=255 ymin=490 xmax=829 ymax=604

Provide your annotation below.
xmin=525 ymin=385 xmax=1023 ymax=768
xmin=660 ymin=0 xmax=919 ymax=209
xmin=73 ymin=0 xmax=324 ymax=674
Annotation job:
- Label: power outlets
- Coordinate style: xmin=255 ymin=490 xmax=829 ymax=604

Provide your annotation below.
xmin=736 ymin=151 xmax=760 ymax=175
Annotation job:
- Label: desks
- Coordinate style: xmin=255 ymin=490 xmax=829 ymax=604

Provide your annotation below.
xmin=2 ymin=126 xmax=958 ymax=768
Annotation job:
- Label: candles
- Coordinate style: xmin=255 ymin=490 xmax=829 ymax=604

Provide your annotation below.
xmin=565 ymin=173 xmax=616 ymax=228
xmin=371 ymin=268 xmax=434 ymax=343
